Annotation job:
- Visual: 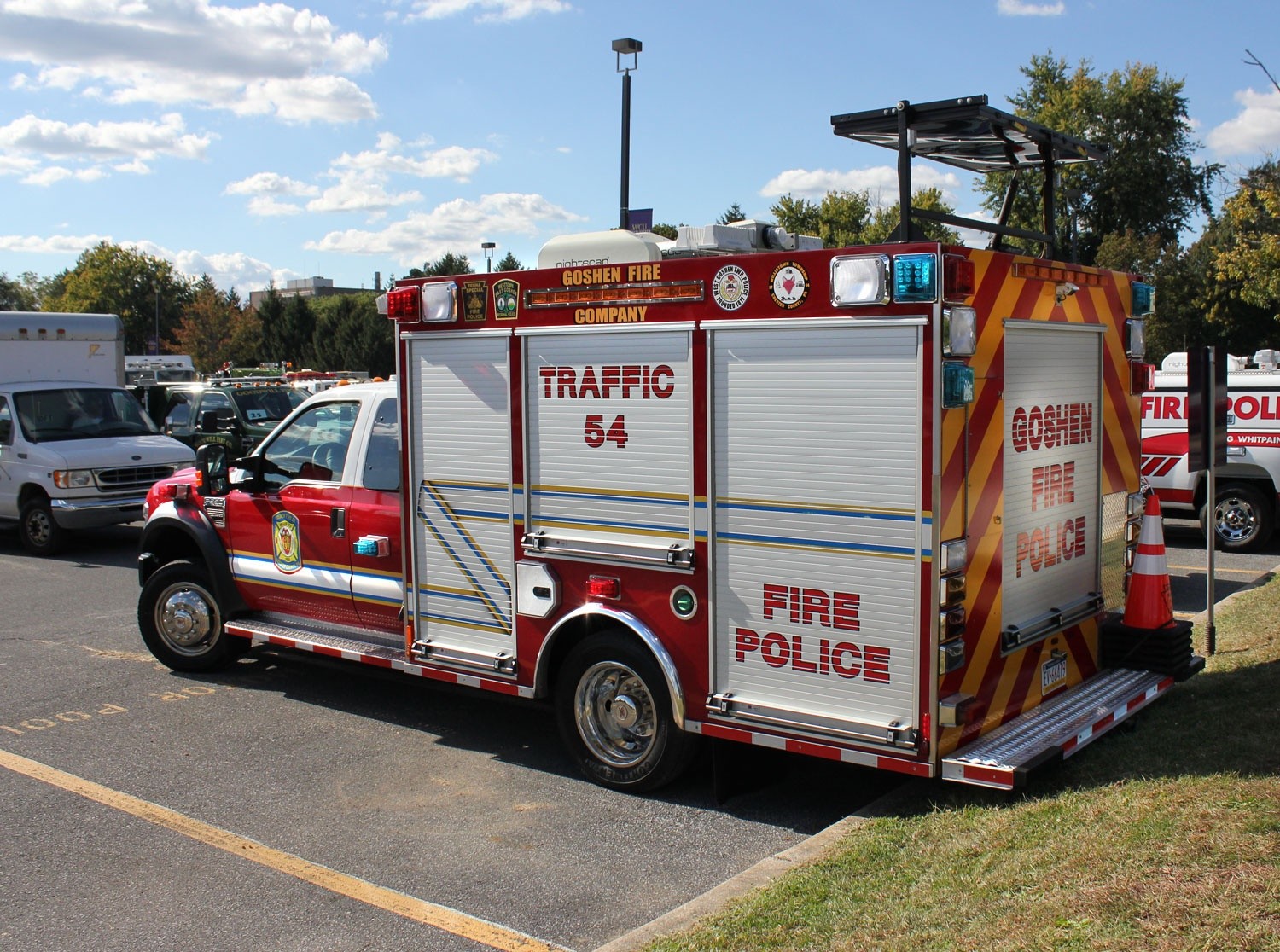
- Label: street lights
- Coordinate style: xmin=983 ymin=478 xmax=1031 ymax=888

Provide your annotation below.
xmin=611 ymin=36 xmax=643 ymax=236
xmin=480 ymin=242 xmax=496 ymax=273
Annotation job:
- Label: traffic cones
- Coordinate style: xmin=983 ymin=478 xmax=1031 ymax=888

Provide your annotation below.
xmin=1108 ymin=493 xmax=1181 ymax=635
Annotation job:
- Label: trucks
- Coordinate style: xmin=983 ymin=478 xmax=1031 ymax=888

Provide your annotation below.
xmin=136 ymin=90 xmax=1227 ymax=793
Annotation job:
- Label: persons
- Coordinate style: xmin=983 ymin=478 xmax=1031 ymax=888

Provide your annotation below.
xmin=69 ymin=394 xmax=111 ymax=429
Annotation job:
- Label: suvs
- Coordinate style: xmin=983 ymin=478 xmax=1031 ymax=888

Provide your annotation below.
xmin=213 ymin=361 xmax=369 ymax=422
xmin=130 ymin=375 xmax=355 ymax=480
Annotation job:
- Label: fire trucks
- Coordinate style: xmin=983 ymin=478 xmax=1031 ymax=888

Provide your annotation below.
xmin=125 ymin=354 xmax=199 ymax=385
xmin=1145 ymin=349 xmax=1280 ymax=552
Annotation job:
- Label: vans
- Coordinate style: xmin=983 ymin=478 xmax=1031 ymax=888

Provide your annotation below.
xmin=0 ymin=311 xmax=195 ymax=558
